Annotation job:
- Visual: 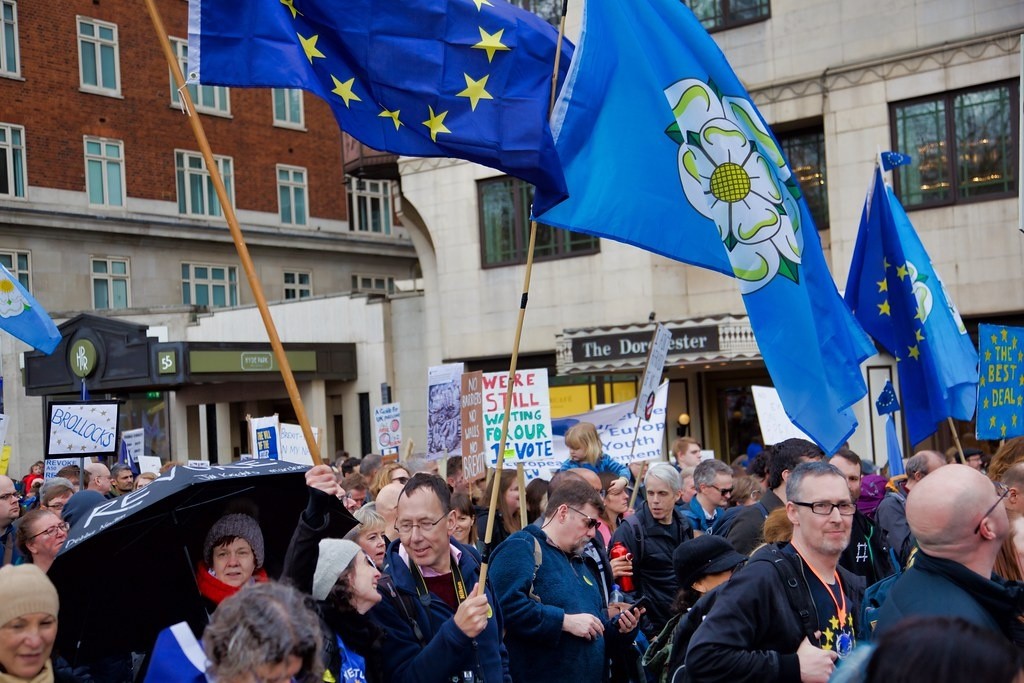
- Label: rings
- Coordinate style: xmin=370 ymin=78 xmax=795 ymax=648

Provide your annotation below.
xmin=627 ymin=626 xmax=631 ymax=629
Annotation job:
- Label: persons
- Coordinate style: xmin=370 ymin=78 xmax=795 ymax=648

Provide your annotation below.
xmin=0 ymin=421 xmax=1024 ymax=683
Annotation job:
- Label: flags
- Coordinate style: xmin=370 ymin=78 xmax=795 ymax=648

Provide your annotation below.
xmin=0 ymin=263 xmax=62 ymax=355
xmin=188 ymin=0 xmax=578 ymax=219
xmin=119 ymin=438 xmax=139 ymax=476
xmin=531 ymin=0 xmax=878 ymax=458
xmin=849 ymin=170 xmax=981 ymax=449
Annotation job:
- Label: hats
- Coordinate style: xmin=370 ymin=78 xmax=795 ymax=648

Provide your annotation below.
xmin=955 ymin=449 xmax=983 ymax=464
xmin=0 ymin=563 xmax=60 ymax=630
xmin=39 ymin=479 xmax=76 ymax=505
xmin=311 ymin=538 xmax=363 ymax=603
xmin=203 ymin=513 xmax=265 ymax=574
xmin=673 ymin=535 xmax=748 ymax=590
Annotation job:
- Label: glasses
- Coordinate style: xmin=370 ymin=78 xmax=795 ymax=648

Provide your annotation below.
xmin=974 ymin=481 xmax=1009 ymax=535
xmin=394 ymin=512 xmax=447 ymax=534
xmin=391 ymin=477 xmax=410 ymax=485
xmin=0 ymin=493 xmax=17 ymax=501
xmin=28 ymin=522 xmax=70 ymax=540
xmin=707 ymin=484 xmax=733 ymax=497
xmin=94 ymin=475 xmax=112 ymax=482
xmin=566 ymin=506 xmax=601 ymax=529
xmin=791 ymin=501 xmax=856 ymax=515
xmin=45 ymin=504 xmax=66 ymax=510
xmin=364 ymin=554 xmax=377 ymax=569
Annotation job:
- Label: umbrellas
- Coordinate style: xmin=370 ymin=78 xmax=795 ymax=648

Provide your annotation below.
xmin=44 ymin=458 xmax=366 ymax=663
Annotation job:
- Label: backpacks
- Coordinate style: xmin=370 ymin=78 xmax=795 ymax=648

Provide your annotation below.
xmin=855 ymin=472 xmax=909 ymax=524
xmin=704 ymin=502 xmax=770 ymax=539
xmin=854 ymin=548 xmax=907 ymax=641
xmin=667 ymin=548 xmax=813 ymax=683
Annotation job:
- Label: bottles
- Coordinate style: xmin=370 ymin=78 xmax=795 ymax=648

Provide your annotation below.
xmin=611 ymin=542 xmax=634 ymax=592
xmin=608 ymin=584 xmax=623 ymax=607
